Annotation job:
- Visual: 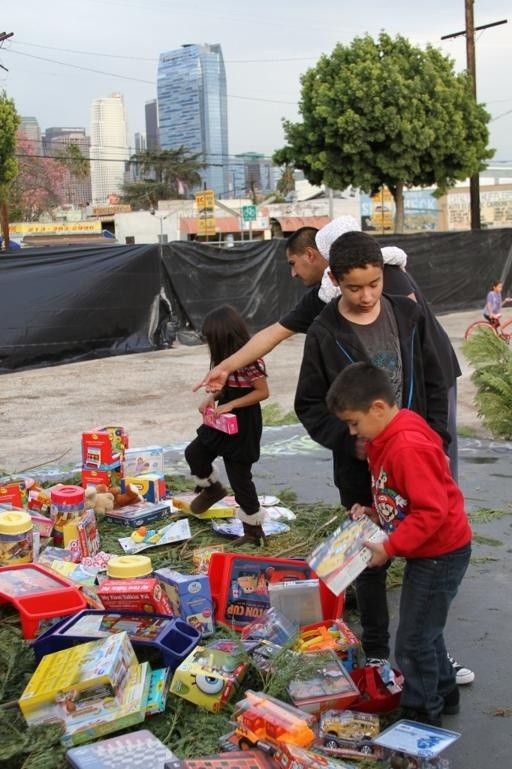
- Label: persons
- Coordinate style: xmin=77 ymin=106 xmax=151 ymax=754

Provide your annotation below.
xmin=192 ymin=226 xmax=436 ymax=394
xmin=481 ymin=278 xmax=504 ymax=328
xmin=182 ymin=304 xmax=270 ymax=548
xmin=295 ymin=232 xmax=473 ymax=684
xmin=325 ymin=358 xmax=474 ymax=727
xmin=315 ymin=216 xmax=407 ymax=304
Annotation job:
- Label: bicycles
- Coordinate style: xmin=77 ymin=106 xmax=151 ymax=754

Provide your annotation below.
xmin=465 ymin=298 xmax=512 ymax=346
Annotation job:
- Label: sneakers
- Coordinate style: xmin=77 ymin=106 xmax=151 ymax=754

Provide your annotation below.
xmin=365 ymin=657 xmax=388 ymax=666
xmin=447 ymin=652 xmax=475 ymax=685
xmin=397 ymin=687 xmax=459 ymax=726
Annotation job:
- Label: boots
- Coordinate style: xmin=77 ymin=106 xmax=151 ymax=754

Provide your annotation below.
xmin=231 ymin=507 xmax=266 ymax=546
xmin=190 ymin=463 xmax=227 ymax=514
